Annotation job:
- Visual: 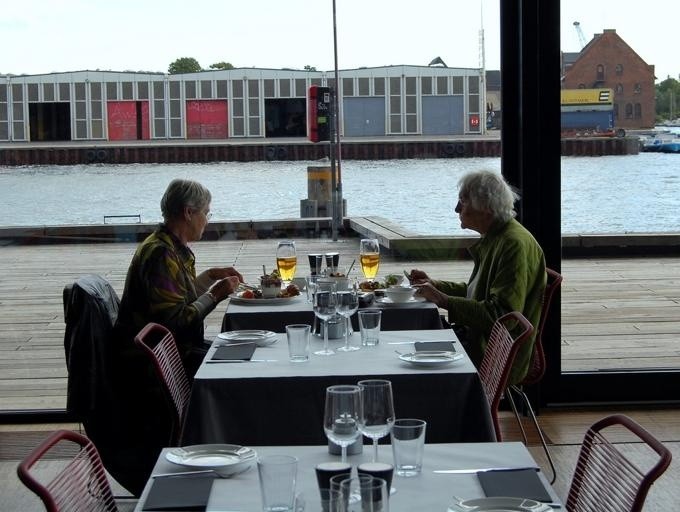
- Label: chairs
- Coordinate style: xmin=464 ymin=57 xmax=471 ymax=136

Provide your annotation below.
xmin=62 ymin=275 xmax=122 ymax=464
xmin=473 ymin=311 xmax=535 ymax=439
xmin=18 ymin=429 xmax=119 ymax=512
xmin=134 ymin=319 xmax=189 ymax=447
xmin=563 ymin=413 xmax=675 ymax=510
xmin=481 ymin=267 xmax=563 ymax=446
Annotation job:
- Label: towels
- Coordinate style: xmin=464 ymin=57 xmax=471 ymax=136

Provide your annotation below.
xmin=477 ymin=469 xmax=553 ymax=504
xmin=414 ymin=342 xmax=456 ymax=356
xmin=142 ymin=469 xmax=216 ymax=512
xmin=211 ymin=343 xmax=258 ymax=361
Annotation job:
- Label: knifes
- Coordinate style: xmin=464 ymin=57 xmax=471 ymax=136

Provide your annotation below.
xmin=206 ymin=359 xmax=279 ymax=363
xmin=433 ymin=466 xmax=542 ymax=473
xmin=387 ymin=340 xmax=456 ymax=345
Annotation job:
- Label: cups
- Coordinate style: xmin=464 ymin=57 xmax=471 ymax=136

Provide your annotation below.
xmin=389 ymin=418 xmax=427 ymax=477
xmin=340 ymin=476 xmax=388 ymax=512
xmin=325 ymin=252 xmax=339 ymax=275
xmin=315 ymin=256 xmax=334 ymax=276
xmin=358 ymin=310 xmax=382 ymax=345
xmin=316 ymin=462 xmax=352 ymax=499
xmin=329 ymin=473 xmax=374 ymax=511
xmin=358 ymin=464 xmax=392 ymax=501
xmin=257 ymin=453 xmax=299 ymax=512
xmin=384 ymin=274 xmax=404 ymax=286
xmin=275 ymin=241 xmax=297 ymax=284
xmin=307 ymin=254 xmax=322 ymax=275
xmin=286 ymin=324 xmax=311 ymax=362
xmin=359 ymin=239 xmax=380 ymax=279
xmin=294 ymin=488 xmax=344 ymax=512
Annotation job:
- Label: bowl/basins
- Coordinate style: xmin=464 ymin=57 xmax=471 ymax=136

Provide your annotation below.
xmin=386 ymin=287 xmax=417 ymax=303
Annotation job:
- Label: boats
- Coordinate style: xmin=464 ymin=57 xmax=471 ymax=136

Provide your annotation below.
xmin=642 ymin=139 xmax=663 ymax=151
xmin=664 ymin=134 xmax=680 ymax=154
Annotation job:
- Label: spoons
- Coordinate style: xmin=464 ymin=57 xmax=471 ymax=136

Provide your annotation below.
xmin=214 ymin=336 xmax=280 ymax=347
xmin=152 ymin=465 xmax=251 ymax=478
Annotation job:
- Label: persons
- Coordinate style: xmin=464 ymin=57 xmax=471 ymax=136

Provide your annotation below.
xmin=410 ymin=170 xmax=547 ymax=401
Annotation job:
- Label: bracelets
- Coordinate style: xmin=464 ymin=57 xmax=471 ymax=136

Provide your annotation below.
xmin=205 ymin=292 xmax=217 ymax=303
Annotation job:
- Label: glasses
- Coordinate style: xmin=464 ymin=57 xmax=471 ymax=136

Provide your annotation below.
xmin=192 ymin=211 xmax=212 ymax=220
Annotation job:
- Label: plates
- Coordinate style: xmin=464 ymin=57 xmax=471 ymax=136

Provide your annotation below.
xmin=218 ymin=330 xmax=276 ymax=343
xmin=165 ymin=443 xmax=257 ymax=468
xmin=228 ymin=289 xmax=303 ymax=304
xmin=375 ymin=296 xmax=426 ymax=307
xmin=398 ymin=351 xmax=464 ymax=367
xmin=447 ymin=495 xmax=554 ymax=512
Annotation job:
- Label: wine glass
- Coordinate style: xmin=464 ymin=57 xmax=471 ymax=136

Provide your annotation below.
xmin=323 ymin=384 xmax=364 ymax=464
xmin=332 ymin=279 xmax=360 ymax=352
xmin=312 ymin=279 xmax=338 ymax=355
xmin=354 ymin=379 xmax=396 ymax=464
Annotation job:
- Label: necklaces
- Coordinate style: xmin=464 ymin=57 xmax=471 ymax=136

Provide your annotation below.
xmin=111 ymin=178 xmax=244 ymax=393
